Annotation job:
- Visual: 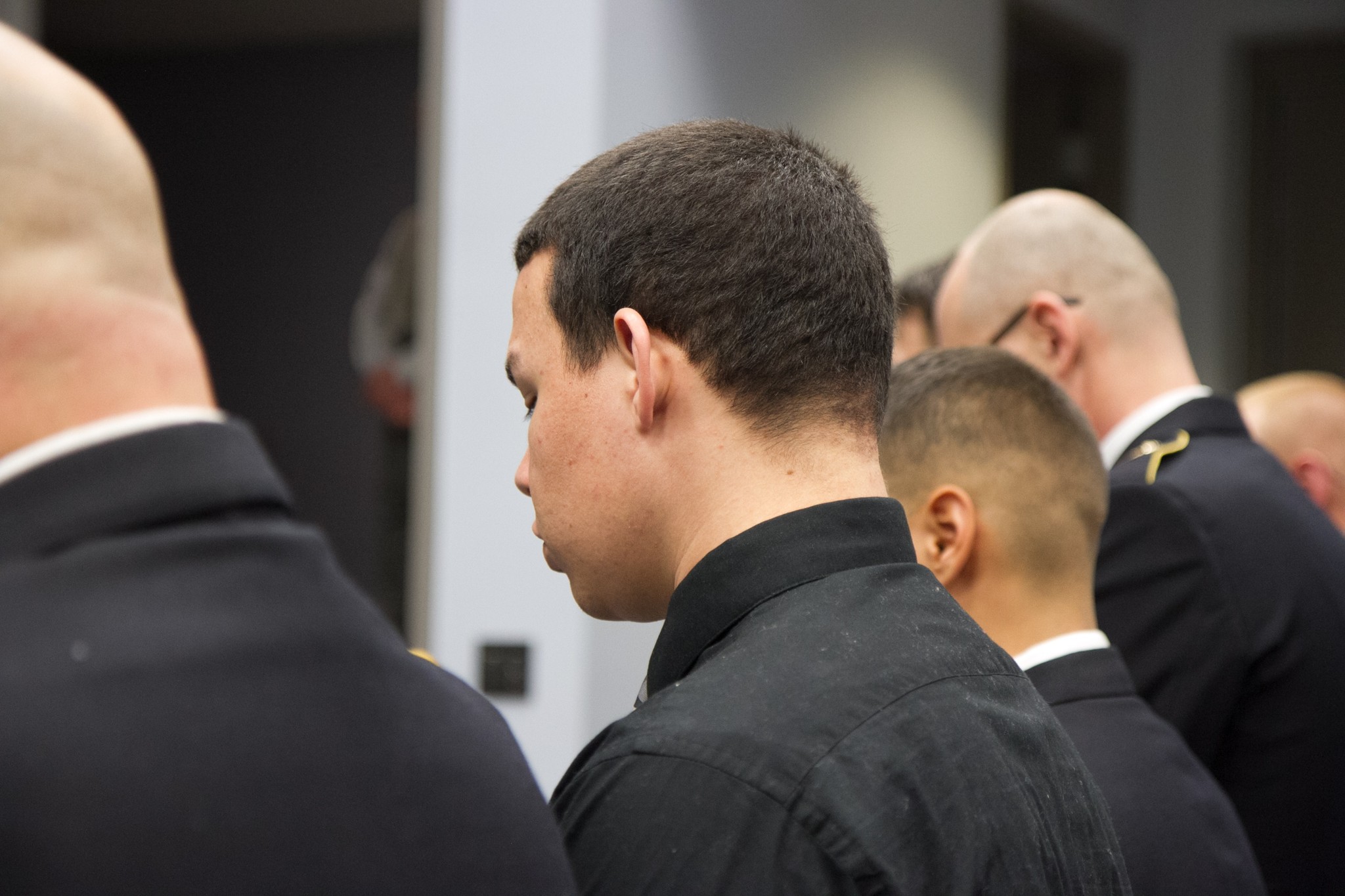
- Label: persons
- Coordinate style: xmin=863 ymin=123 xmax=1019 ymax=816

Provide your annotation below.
xmin=891 ymin=255 xmax=956 ymax=369
xmin=934 ymin=189 xmax=1345 ymax=896
xmin=877 ymin=343 xmax=1265 ymax=896
xmin=503 ymin=118 xmax=1132 ymax=896
xmin=1237 ymin=370 xmax=1345 ymax=532
xmin=0 ymin=19 xmax=576 ymax=896
